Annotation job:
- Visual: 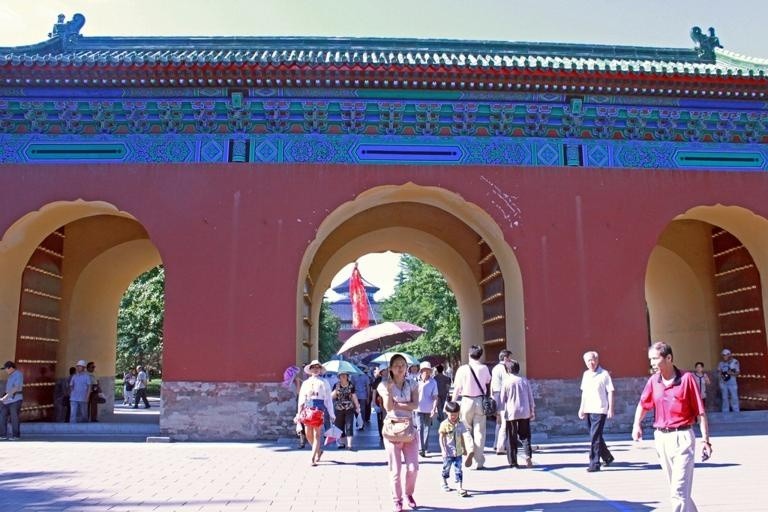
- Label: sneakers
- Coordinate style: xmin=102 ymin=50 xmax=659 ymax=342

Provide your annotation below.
xmin=406 ymin=496 xmax=416 ymax=508
xmin=465 ymin=450 xmax=474 ymax=466
xmin=418 ymin=450 xmax=426 ymax=458
xmin=395 ymin=502 xmax=402 ymax=512
xmin=526 ymin=456 xmax=531 ymax=468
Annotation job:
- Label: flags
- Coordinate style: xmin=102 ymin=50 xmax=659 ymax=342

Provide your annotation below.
xmin=349 ymin=269 xmax=369 ymax=330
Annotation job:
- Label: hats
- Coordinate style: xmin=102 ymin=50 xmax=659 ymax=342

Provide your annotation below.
xmin=76 ymin=360 xmax=86 ymax=368
xmin=303 ymin=361 xmax=434 ymax=379
xmin=720 ymin=349 xmax=730 ymax=355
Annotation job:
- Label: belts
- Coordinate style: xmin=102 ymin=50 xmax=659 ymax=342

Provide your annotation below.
xmin=658 ymin=425 xmax=691 ymax=433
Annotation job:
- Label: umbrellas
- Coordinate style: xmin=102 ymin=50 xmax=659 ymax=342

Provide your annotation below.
xmin=336 ymin=321 xmax=429 ymax=378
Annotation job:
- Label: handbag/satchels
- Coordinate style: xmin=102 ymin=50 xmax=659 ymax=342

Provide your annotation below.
xmin=300 ymin=408 xmax=325 ymax=429
xmin=483 ymin=397 xmax=497 ymax=416
xmin=382 ymin=414 xmax=417 ymax=443
xmin=721 ymin=372 xmax=730 ymax=382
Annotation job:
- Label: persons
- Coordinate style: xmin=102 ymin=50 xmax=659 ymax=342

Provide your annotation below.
xmin=0 ymin=359 xmax=150 ymax=441
xmin=297 ymin=349 xmax=536 ymax=512
xmin=577 ymin=351 xmax=616 ymax=472
xmin=632 ymin=341 xmax=741 ymax=512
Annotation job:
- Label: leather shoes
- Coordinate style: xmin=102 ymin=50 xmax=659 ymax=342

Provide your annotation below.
xmin=603 ymin=455 xmax=614 ymax=466
xmin=587 ymin=466 xmax=599 ymax=471
